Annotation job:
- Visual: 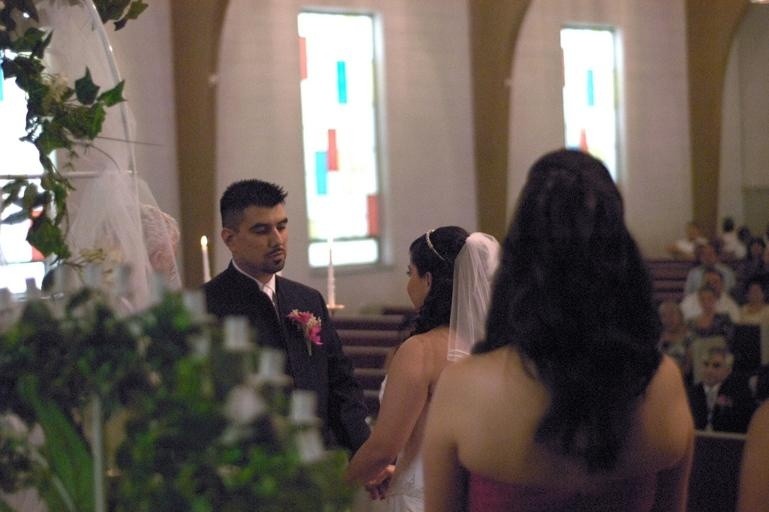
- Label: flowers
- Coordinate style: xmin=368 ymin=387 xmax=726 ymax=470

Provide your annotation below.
xmin=287 ymin=306 xmax=325 ymax=348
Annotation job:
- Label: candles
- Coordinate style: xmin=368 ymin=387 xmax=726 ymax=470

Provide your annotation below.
xmin=200 ymin=245 xmax=212 ymax=283
xmin=327 ymin=249 xmax=336 ymax=305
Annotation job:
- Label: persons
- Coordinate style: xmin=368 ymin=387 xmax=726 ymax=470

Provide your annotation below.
xmin=655 ymin=213 xmax=769 ymax=512
xmin=342 ymin=225 xmax=502 ymax=512
xmin=189 ymin=177 xmax=397 ymax=502
xmin=420 ymin=149 xmax=695 ymax=512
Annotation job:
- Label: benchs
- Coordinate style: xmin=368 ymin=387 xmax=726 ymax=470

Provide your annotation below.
xmin=328 ymin=253 xmax=755 ymax=493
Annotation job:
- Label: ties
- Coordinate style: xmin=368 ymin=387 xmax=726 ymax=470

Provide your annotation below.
xmin=263 ymin=286 xmax=278 ymax=318
